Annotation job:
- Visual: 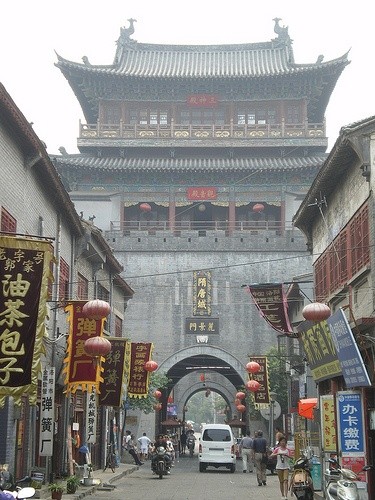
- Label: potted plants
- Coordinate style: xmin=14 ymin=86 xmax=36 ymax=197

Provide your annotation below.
xmin=31 ymin=480 xmax=42 ymax=499
xmin=63 ymin=475 xmax=80 ymax=494
xmin=84 ymin=464 xmax=94 ymax=486
xmin=46 ymin=481 xmax=67 ymax=500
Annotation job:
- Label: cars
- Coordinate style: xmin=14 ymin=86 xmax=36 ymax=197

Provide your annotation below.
xmin=235 ymin=438 xmax=243 ymax=459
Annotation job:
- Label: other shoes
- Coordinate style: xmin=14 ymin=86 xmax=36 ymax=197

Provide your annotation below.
xmin=284 ymin=497 xmax=289 ymax=500
xmin=263 ymin=481 xmax=266 ymax=485
xmin=168 ymin=471 xmax=170 ymax=473
xmin=140 ymin=463 xmax=144 ymax=466
xmin=258 ymin=483 xmax=262 ymax=486
xmin=281 ymin=496 xmax=285 ymax=500
xmin=152 ymin=472 xmax=155 ymax=475
xmin=251 ymin=471 xmax=253 ymax=473
xmin=243 ymin=470 xmax=247 ymax=473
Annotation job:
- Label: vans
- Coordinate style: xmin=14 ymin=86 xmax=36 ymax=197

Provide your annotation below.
xmin=198 ymin=424 xmax=238 ymax=473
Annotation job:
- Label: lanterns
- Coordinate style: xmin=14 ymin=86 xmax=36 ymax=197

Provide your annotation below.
xmin=154 ymin=405 xmax=161 ymax=410
xmin=144 ymin=361 xmax=158 ymax=373
xmin=237 ymin=405 xmax=246 ymax=412
xmin=245 ymin=362 xmax=260 ymax=373
xmin=141 ymin=203 xmax=151 ymax=212
xmin=247 ymin=380 xmax=260 ymax=391
xmin=253 ymin=204 xmax=264 ymax=212
xmin=83 ymin=299 xmax=111 ymax=318
xmin=236 ymin=392 xmax=245 ymax=399
xmin=84 ymin=337 xmax=112 ymax=357
xmin=153 ymin=391 xmax=161 ymax=398
xmin=302 ymin=303 xmax=331 ymax=322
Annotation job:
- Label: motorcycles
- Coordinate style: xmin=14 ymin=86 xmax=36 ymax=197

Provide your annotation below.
xmin=151 ymin=444 xmax=174 ymax=479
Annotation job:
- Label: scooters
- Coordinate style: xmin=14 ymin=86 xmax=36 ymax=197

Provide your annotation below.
xmin=289 ymin=455 xmax=315 ymax=500
xmin=324 ymin=457 xmax=372 ymax=500
xmin=266 ymin=446 xmax=278 ymax=475
xmin=187 ymin=438 xmax=197 ymax=455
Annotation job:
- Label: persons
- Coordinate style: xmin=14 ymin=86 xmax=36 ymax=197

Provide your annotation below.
xmin=252 ymin=430 xmax=267 ymax=485
xmin=138 ymin=433 xmax=151 ymax=460
xmin=271 ymin=437 xmax=290 ymax=500
xmin=275 ymin=427 xmax=283 ymax=445
xmin=0 ymin=485 xmax=15 ymax=500
xmin=239 ymin=432 xmax=253 ymax=472
xmin=124 ymin=432 xmax=145 ymax=466
xmin=152 ymin=430 xmax=196 ymax=474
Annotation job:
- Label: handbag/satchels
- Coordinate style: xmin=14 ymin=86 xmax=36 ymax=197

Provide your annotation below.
xmin=262 ymin=455 xmax=268 ymax=463
